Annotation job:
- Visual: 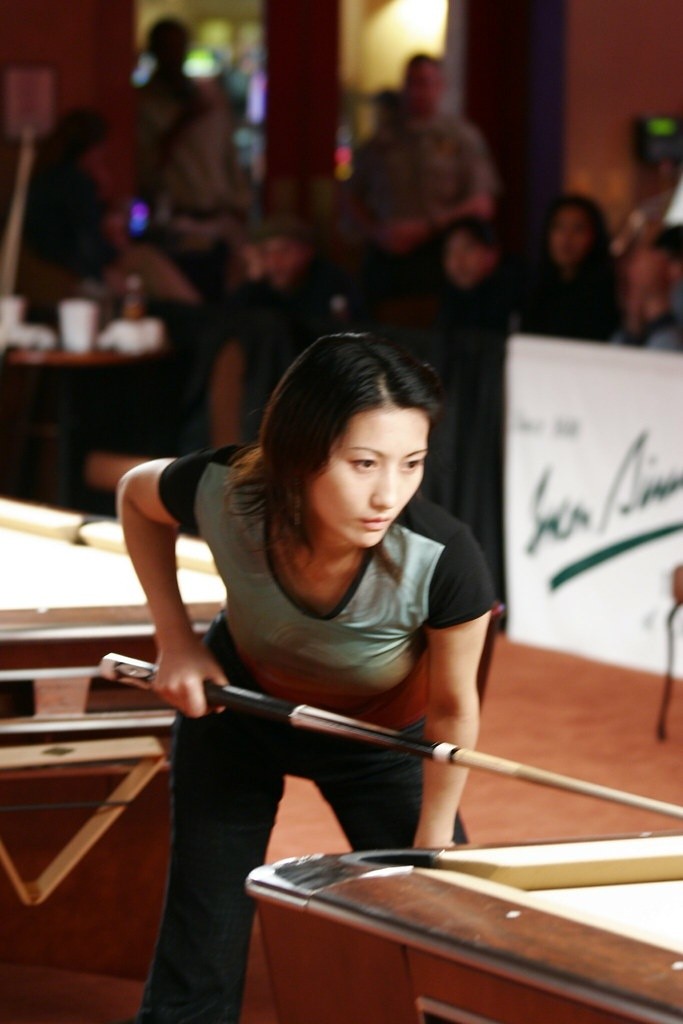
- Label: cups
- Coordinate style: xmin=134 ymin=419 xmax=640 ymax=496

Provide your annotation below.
xmin=59 ymin=299 xmax=99 ymax=352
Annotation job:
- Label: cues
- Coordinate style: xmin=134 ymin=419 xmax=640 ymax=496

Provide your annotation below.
xmin=102 ymin=652 xmax=683 ymax=819
xmin=1 ymin=127 xmax=38 ymax=295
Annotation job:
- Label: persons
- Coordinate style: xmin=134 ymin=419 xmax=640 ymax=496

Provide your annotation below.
xmin=17 ymin=13 xmax=683 ymax=450
xmin=119 ymin=331 xmax=498 ymax=1024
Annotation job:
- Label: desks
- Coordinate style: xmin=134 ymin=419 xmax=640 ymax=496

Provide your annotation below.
xmin=0 ymin=496 xmax=504 ymax=982
xmin=244 ymin=830 xmax=683 ymax=1024
xmin=0 ymin=327 xmax=178 ymax=510
